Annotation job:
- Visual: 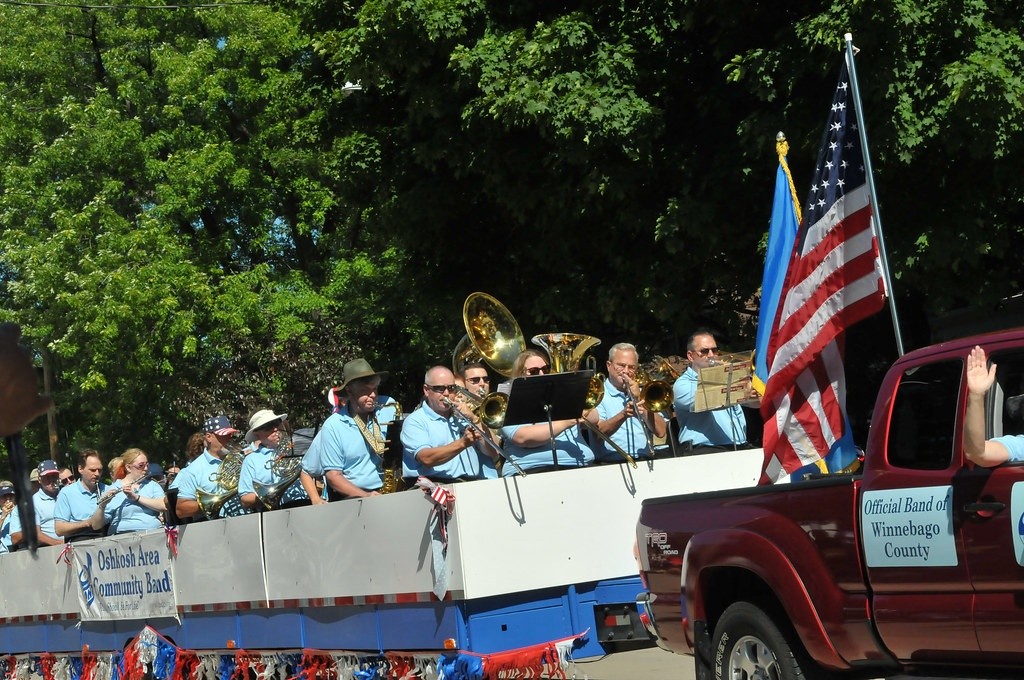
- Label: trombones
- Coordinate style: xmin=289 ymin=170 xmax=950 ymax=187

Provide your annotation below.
xmin=620 ymin=363 xmax=675 ymax=457
xmin=578 ymin=376 xmax=639 ymax=470
xmin=441 ymin=384 xmax=528 ymax=479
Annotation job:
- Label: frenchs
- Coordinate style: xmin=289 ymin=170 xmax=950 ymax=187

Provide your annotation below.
xmin=452 ymin=292 xmax=526 ymax=378
xmin=252 ymin=436 xmax=303 ymax=511
xmin=194 ymin=440 xmax=245 ymax=521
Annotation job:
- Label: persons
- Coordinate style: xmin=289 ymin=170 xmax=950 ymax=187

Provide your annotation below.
xmin=300 ymin=358 xmax=405 ymax=506
xmin=962 ymin=345 xmax=1024 ymax=467
xmin=672 ymin=332 xmax=760 ymax=456
xmin=400 ymin=343 xmax=678 ymax=491
xmin=0 ymin=411 xmax=330 ymax=558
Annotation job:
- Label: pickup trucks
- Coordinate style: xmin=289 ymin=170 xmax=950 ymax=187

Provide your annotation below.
xmin=632 ymin=323 xmax=1024 ymax=680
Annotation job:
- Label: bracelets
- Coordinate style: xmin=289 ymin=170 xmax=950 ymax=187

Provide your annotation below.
xmin=134 ymin=495 xmax=140 ymax=502
xmin=99 ymin=505 xmax=106 ymax=509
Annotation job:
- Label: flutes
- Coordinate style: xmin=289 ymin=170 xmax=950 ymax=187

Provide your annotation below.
xmin=95 ymin=475 xmax=149 ymax=505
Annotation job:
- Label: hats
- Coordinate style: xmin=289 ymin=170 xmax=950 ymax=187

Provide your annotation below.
xmin=30 ymin=468 xmax=40 ymax=482
xmin=332 ymin=356 xmax=392 ymax=397
xmin=201 ymin=415 xmax=241 ymax=438
xmin=243 ymin=409 xmax=288 ymax=441
xmin=0 ymin=486 xmax=16 ymax=495
xmin=148 ymin=463 xmax=164 ymax=476
xmin=37 ymin=459 xmax=60 ymax=476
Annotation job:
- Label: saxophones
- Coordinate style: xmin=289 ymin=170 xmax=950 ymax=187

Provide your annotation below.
xmin=373 ymin=401 xmax=408 ymax=494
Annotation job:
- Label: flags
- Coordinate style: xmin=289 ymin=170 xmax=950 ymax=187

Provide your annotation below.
xmin=756 ymin=56 xmax=890 ymax=487
xmin=751 ymin=143 xmax=861 ymax=483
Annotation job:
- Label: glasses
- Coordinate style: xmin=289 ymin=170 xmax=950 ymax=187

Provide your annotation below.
xmin=524 ymin=364 xmax=552 ymax=375
xmin=425 ymin=383 xmax=458 ymax=394
xmin=691 ymin=346 xmax=719 ymax=357
xmin=61 ymin=475 xmax=75 ymax=483
xmin=464 ymin=376 xmax=491 ymax=384
xmin=129 ymin=462 xmax=150 ymax=470
xmin=610 ymin=362 xmax=638 ymax=374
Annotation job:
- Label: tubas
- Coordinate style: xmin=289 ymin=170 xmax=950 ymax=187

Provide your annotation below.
xmin=531 ymin=332 xmax=601 ymax=374
